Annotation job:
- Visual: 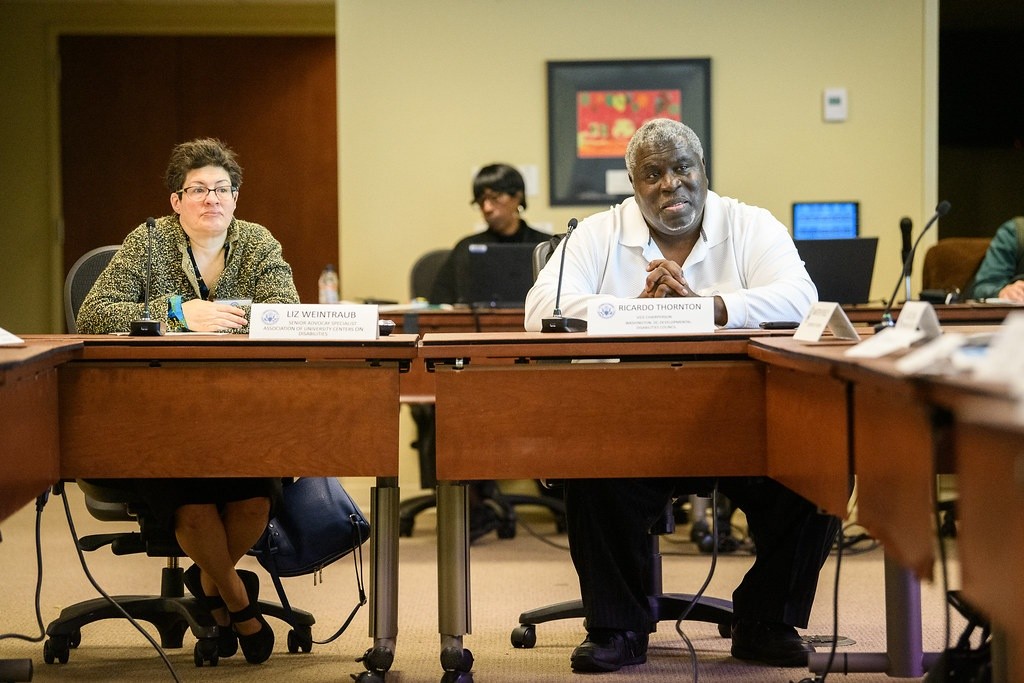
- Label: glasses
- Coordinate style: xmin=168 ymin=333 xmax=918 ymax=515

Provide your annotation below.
xmin=176 ymin=185 xmax=237 ymax=200
xmin=471 ymin=192 xmax=503 ymax=209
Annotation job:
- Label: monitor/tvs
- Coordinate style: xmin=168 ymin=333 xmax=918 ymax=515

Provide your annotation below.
xmin=467 ymin=243 xmax=540 ymax=308
xmin=792 ymin=238 xmax=878 ymax=304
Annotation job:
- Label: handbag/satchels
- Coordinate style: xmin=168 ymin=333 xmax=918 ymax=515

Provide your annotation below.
xmin=257 ymin=476 xmax=371 ymax=643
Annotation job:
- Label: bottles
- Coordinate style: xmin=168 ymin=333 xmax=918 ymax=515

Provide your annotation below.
xmin=318 ymin=263 xmax=338 ymax=303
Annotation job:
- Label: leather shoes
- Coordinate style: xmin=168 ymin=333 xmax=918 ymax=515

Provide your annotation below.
xmin=571 ymin=629 xmax=649 ymax=670
xmin=731 ymin=619 xmax=816 ymax=665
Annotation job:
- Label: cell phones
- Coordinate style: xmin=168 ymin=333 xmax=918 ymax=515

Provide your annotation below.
xmin=759 ymin=322 xmax=800 ymax=329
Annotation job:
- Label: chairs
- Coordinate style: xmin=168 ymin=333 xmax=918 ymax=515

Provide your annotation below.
xmin=511 ymin=242 xmax=732 ymax=648
xmin=390 ymin=245 xmax=566 ymax=540
xmin=923 ymin=231 xmax=1001 ymax=300
xmin=39 ymin=246 xmax=316 ymax=665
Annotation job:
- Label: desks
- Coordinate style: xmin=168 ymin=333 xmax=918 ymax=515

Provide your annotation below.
xmin=0 ymin=297 xmax=1024 ymax=683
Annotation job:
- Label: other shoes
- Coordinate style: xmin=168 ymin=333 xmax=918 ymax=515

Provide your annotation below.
xmin=183 ymin=563 xmax=238 ymax=658
xmin=939 ymin=521 xmax=956 ymax=537
xmin=230 ymin=569 xmax=274 ymax=664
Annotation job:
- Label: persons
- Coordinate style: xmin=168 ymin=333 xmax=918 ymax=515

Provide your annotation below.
xmin=428 ymin=164 xmax=552 ymax=531
xmin=687 ymin=493 xmax=715 ymax=552
xmin=972 ymin=216 xmax=1024 ymax=302
xmin=76 ymin=138 xmax=302 ymax=665
xmin=524 ymin=118 xmax=842 ymax=672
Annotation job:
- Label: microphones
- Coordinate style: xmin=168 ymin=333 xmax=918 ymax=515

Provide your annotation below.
xmin=128 ymin=217 xmax=163 ymax=336
xmin=541 ymin=218 xmax=587 ymax=333
xmin=873 ymin=201 xmax=951 ymax=334
xmin=900 ymin=218 xmax=913 ymax=276
